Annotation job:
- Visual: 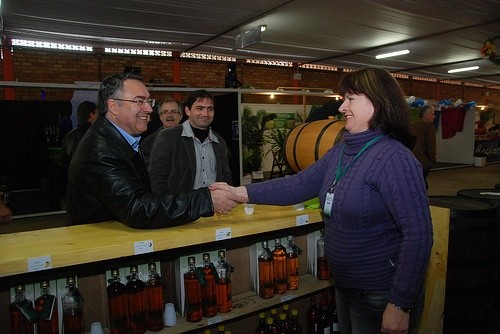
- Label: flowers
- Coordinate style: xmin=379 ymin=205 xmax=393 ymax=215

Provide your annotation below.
xmin=481 ymin=34 xmax=500 ymax=70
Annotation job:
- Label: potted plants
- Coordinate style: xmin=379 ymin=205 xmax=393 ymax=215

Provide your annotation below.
xmin=241 ymin=104 xmax=277 ymax=184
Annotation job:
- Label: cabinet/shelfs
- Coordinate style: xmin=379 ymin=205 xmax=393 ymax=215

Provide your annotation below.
xmin=0 ymin=205 xmax=336 ymax=334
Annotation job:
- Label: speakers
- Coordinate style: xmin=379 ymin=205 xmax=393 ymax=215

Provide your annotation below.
xmin=225 ymin=74 xmax=238 ymax=88
xmin=123 ymin=67 xmax=142 ymax=75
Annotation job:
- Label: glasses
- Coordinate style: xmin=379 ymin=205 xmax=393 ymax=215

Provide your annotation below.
xmin=159 ymin=109 xmax=181 ymax=116
xmin=106 ymin=97 xmax=155 ymax=109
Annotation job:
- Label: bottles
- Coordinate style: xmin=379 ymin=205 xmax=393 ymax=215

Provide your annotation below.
xmin=61 ymin=277 xmax=85 ymax=334
xmin=258 ymin=241 xmax=274 ymax=299
xmin=307 ymin=291 xmax=339 ymax=334
xmin=255 ymin=304 xmax=302 ymax=334
xmin=218 ymin=325 xmax=232 ymax=334
xmin=126 ymin=266 xmax=146 ymax=334
xmin=273 ymin=238 xmax=287 ymax=294
xmin=107 ymin=268 xmax=126 ymax=334
xmin=184 ymin=257 xmax=202 ymax=322
xmin=35 ymin=281 xmax=60 ymax=334
xmin=215 ymin=251 xmax=233 ymax=313
xmin=204 ymin=329 xmax=211 ymax=334
xmin=316 ymin=236 xmax=330 ymax=280
xmin=200 ymin=253 xmax=217 ymax=317
xmin=146 ymin=263 xmax=164 ymax=331
xmin=286 ymin=235 xmax=300 ymax=291
xmin=9 ymin=284 xmax=34 ymax=334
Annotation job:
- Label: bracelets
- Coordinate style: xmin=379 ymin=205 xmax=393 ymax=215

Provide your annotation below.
xmin=218 ymin=211 xmax=221 ymax=215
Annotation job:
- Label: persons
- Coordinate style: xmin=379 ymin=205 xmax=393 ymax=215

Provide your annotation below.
xmin=61 ymin=101 xmax=98 ymax=166
xmin=208 ymin=66 xmax=433 ymax=334
xmin=409 ymin=106 xmax=435 ymax=190
xmin=141 ymin=99 xmax=183 ymax=162
xmin=67 ymin=75 xmax=246 ymax=229
xmin=149 ymin=90 xmax=233 ymax=195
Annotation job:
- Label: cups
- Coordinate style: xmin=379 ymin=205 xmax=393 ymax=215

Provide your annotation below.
xmin=164 ymin=303 xmax=176 ymax=326
xmin=91 ymin=322 xmax=103 ymax=334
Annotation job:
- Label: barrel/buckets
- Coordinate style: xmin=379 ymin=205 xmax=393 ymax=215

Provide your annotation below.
xmin=427 ymin=195 xmax=498 ymax=334
xmin=456 ymin=189 xmax=500 ymax=334
xmin=283 ymin=116 xmax=346 ymax=173
